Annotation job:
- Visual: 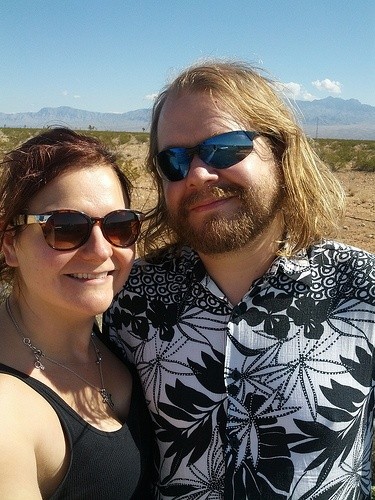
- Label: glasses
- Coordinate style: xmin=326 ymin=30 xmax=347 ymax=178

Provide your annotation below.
xmin=9 ymin=209 xmax=145 ymax=251
xmin=152 ymin=131 xmax=278 ymax=182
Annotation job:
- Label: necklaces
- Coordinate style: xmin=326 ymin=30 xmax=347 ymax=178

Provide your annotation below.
xmin=4 ymin=293 xmax=117 ymax=414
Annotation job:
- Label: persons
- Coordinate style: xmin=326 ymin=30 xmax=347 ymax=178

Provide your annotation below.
xmin=0 ymin=124 xmax=162 ymax=500
xmin=101 ymin=61 xmax=374 ymax=500
